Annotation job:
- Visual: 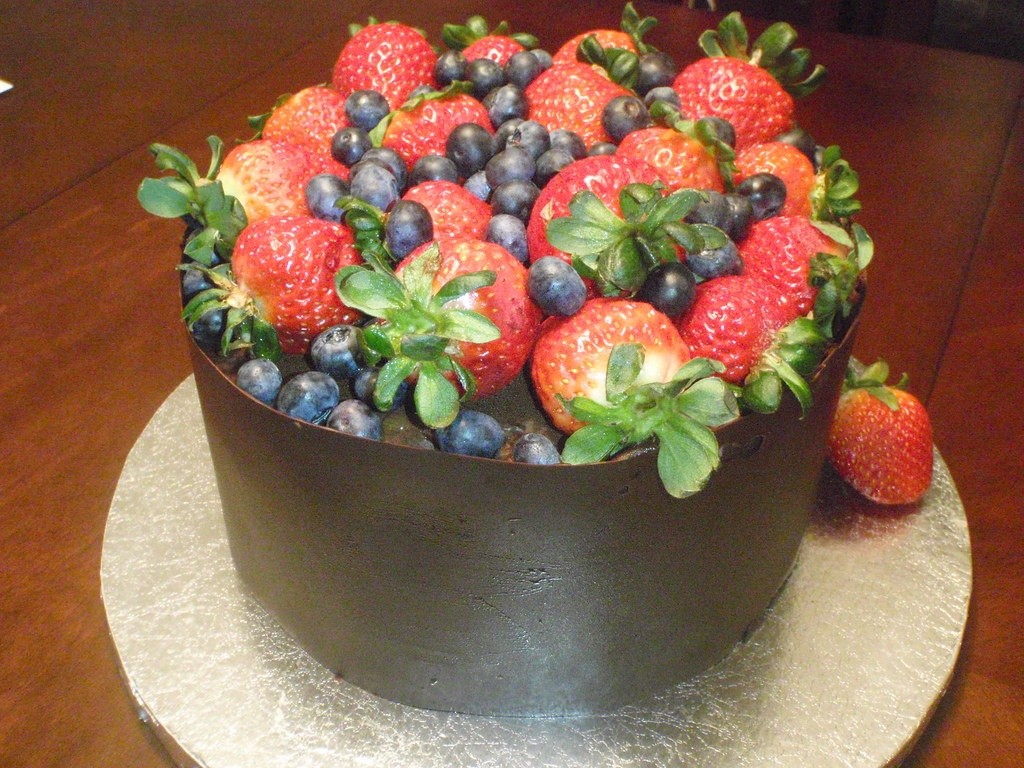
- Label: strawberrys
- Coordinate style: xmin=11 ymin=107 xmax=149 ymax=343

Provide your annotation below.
xmin=135 ymin=2 xmax=878 ymax=497
xmin=824 ymin=354 xmax=933 ymax=504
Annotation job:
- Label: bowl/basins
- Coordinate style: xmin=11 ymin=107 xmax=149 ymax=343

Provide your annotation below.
xmin=182 ymin=219 xmax=868 ymax=717
